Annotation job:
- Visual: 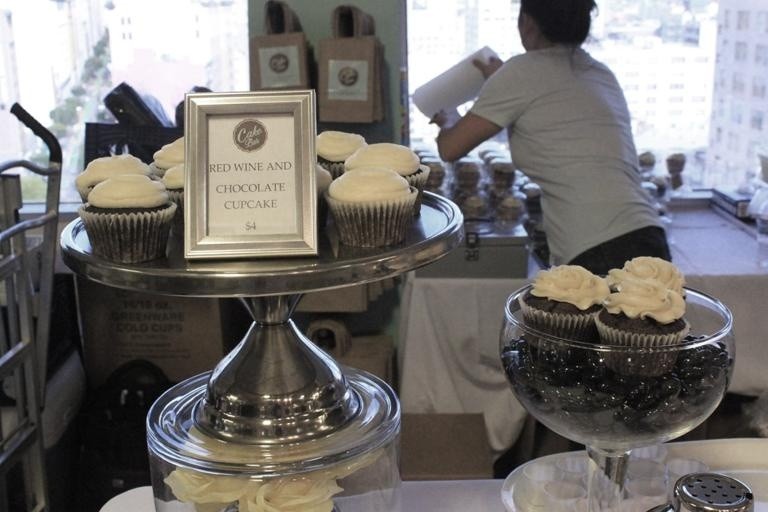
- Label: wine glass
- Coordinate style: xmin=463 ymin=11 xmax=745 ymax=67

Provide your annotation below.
xmin=498 ymin=280 xmax=736 ymax=511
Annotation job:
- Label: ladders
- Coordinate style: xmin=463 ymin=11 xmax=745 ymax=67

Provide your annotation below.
xmin=1 ymin=174 xmax=49 ymax=510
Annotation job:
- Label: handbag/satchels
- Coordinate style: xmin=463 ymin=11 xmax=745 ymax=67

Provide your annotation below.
xmin=305 ymin=318 xmax=394 ymax=394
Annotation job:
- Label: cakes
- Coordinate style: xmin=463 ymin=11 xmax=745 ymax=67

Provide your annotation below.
xmin=75 ymin=134 xmax=187 ymax=213
xmin=163 ymin=467 xmax=344 ymax=512
xmin=523 ymin=254 xmax=688 ymax=335
xmin=315 ymin=130 xmax=423 ymax=202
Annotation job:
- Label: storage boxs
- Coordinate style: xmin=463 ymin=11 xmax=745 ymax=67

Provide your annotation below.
xmin=69 ymin=249 xmax=246 ymax=381
xmin=333 ymin=412 xmax=492 ymax=498
xmin=411 ymin=218 xmax=530 ymax=281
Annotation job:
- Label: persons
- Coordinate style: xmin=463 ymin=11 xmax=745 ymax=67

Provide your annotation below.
xmin=428 ymin=0 xmax=672 ymax=482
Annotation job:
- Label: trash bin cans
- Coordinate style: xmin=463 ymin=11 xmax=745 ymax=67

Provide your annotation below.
xmin=45 ymin=359 xmax=171 ymax=511
